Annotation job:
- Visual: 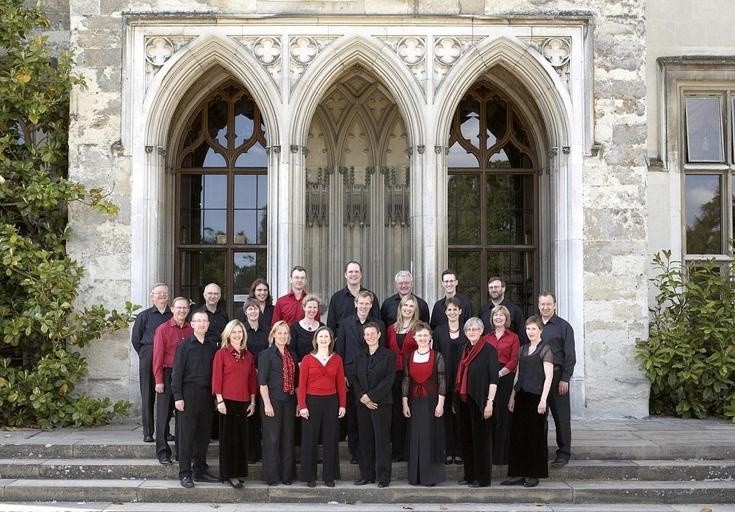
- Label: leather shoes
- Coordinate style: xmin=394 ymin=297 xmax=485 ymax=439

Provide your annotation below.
xmin=194 ymin=473 xmax=221 ymax=482
xmin=158 ymin=450 xmax=172 ymax=464
xmin=354 ymin=479 xmax=375 ymax=485
xmin=166 ymin=433 xmax=175 ymax=441
xmin=551 ymin=456 xmax=568 ymax=469
xmin=144 ymin=435 xmax=153 ymax=442
xmin=445 ymin=455 xmax=463 ymax=465
xmin=181 ymin=472 xmax=194 ymax=488
xmin=308 ymin=480 xmax=316 ymax=487
xmin=351 ymin=453 xmax=359 ymax=464
xmin=500 ymin=477 xmax=539 ymax=487
xmin=268 ymin=480 xmax=291 ymax=486
xmin=325 ymin=480 xmax=335 ymax=487
xmin=378 ymin=480 xmax=390 ymax=488
xmin=458 ymin=479 xmax=490 ymax=487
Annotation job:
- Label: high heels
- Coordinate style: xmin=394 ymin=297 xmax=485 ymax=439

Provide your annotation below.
xmin=221 ymin=477 xmax=245 ymax=488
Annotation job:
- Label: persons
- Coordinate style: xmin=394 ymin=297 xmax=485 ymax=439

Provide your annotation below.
xmin=212 ymin=318 xmax=258 ymax=487
xmin=351 ymin=322 xmax=397 ymax=487
xmin=401 ymin=322 xmax=447 ymax=487
xmin=500 ymin=314 xmax=553 ymax=487
xmin=535 ymin=290 xmax=577 ymax=466
xmin=189 ymin=261 xmax=525 ymax=465
xmin=451 ymin=316 xmax=498 ymax=487
xmin=255 ymin=320 xmax=299 ymax=486
xmin=171 ymin=310 xmax=220 ymax=488
xmin=153 ymin=297 xmax=194 ymax=464
xmin=132 ymin=283 xmax=175 ymax=442
xmin=299 ymin=326 xmax=346 ymax=487
